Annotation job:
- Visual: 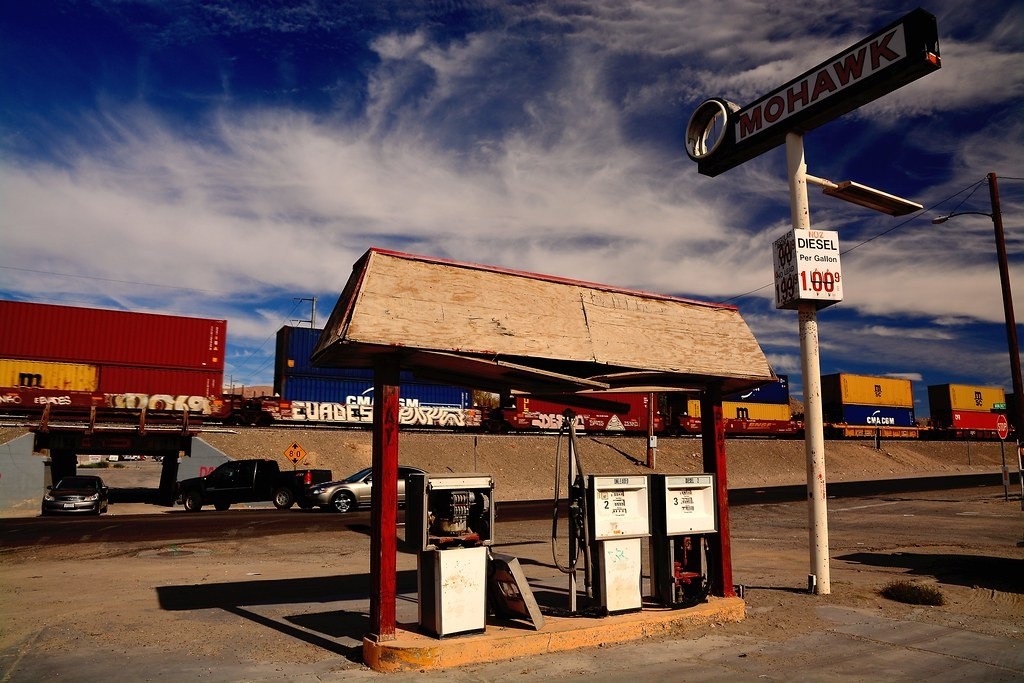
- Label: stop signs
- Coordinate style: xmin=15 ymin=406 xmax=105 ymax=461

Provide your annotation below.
xmin=997 ymin=415 xmax=1008 ymax=439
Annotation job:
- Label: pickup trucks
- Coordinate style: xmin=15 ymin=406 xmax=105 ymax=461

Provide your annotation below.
xmin=180 ymin=459 xmax=332 ymax=513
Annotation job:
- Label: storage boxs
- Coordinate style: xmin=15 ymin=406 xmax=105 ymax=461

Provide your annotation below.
xmin=928 ymin=384 xmax=1007 ymax=438
xmin=0 ymin=300 xmax=227 ymax=417
xmin=821 ymin=373 xmax=916 ymax=437
xmin=488 ymin=390 xmax=667 ymax=433
xmin=676 ymin=375 xmax=791 ymax=439
xmin=273 ymin=325 xmax=473 ymax=426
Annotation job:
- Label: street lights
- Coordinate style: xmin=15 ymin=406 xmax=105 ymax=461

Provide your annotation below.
xmin=931 ymin=211 xmax=1024 ymax=469
xmin=790 ymin=174 xmax=924 ymax=593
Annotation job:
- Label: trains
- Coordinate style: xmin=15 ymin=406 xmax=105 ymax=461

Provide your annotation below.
xmin=0 ymin=298 xmax=1009 ymax=439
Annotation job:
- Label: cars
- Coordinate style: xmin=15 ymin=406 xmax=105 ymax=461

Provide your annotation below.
xmin=41 ymin=475 xmax=109 ymax=516
xmin=309 ymin=465 xmax=429 ymax=514
